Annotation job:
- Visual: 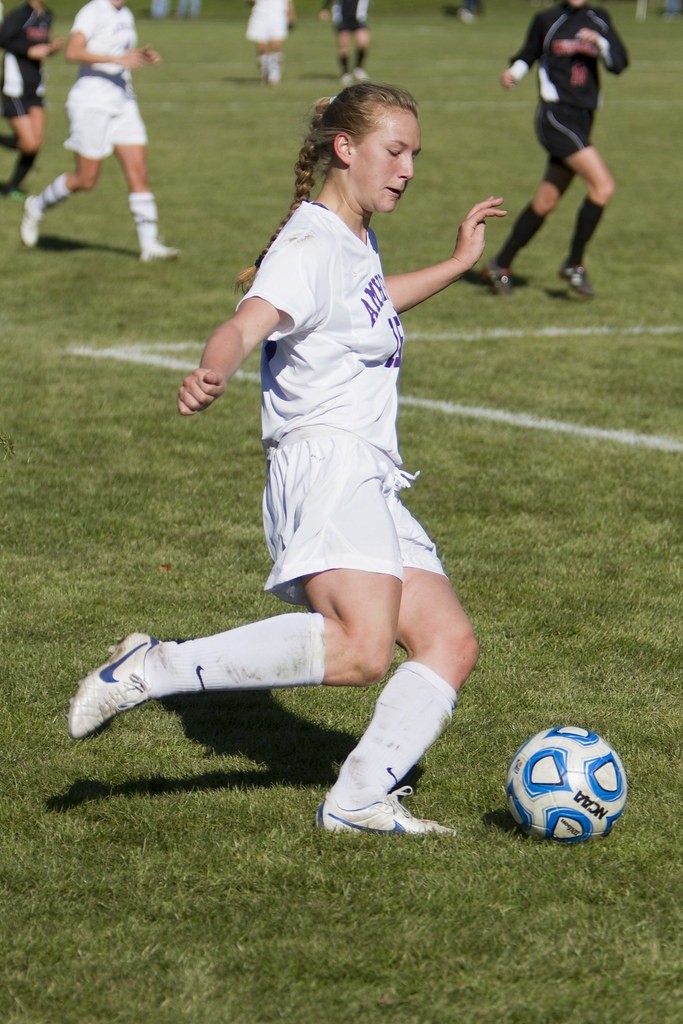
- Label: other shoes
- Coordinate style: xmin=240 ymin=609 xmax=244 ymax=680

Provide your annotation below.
xmin=139 ymin=243 xmax=178 ymax=262
xmin=353 ymin=68 xmax=370 ymax=81
xmin=341 ymin=72 xmax=352 ymax=86
xmin=7 ymin=189 xmax=26 ymax=200
xmin=19 ymin=193 xmax=43 ymax=245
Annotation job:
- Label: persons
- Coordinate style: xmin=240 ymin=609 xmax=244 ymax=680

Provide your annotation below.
xmin=319 ymin=0 xmax=372 ymax=84
xmin=245 ymin=0 xmax=296 ymax=85
xmin=65 ymin=86 xmax=507 ymax=831
xmin=19 ymin=0 xmax=179 ymax=262
xmin=478 ymin=0 xmax=632 ymax=298
xmin=0 ymin=0 xmax=64 ymax=201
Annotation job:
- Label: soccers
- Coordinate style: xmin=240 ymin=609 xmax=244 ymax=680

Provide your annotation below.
xmin=506 ymin=725 xmax=627 ymax=846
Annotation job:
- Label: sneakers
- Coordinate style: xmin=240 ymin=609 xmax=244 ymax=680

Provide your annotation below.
xmin=482 ymin=259 xmax=510 ymax=297
xmin=69 ymin=629 xmax=158 ymax=740
xmin=559 ymin=261 xmax=592 ymax=294
xmin=314 ymin=785 xmax=453 ymax=839
